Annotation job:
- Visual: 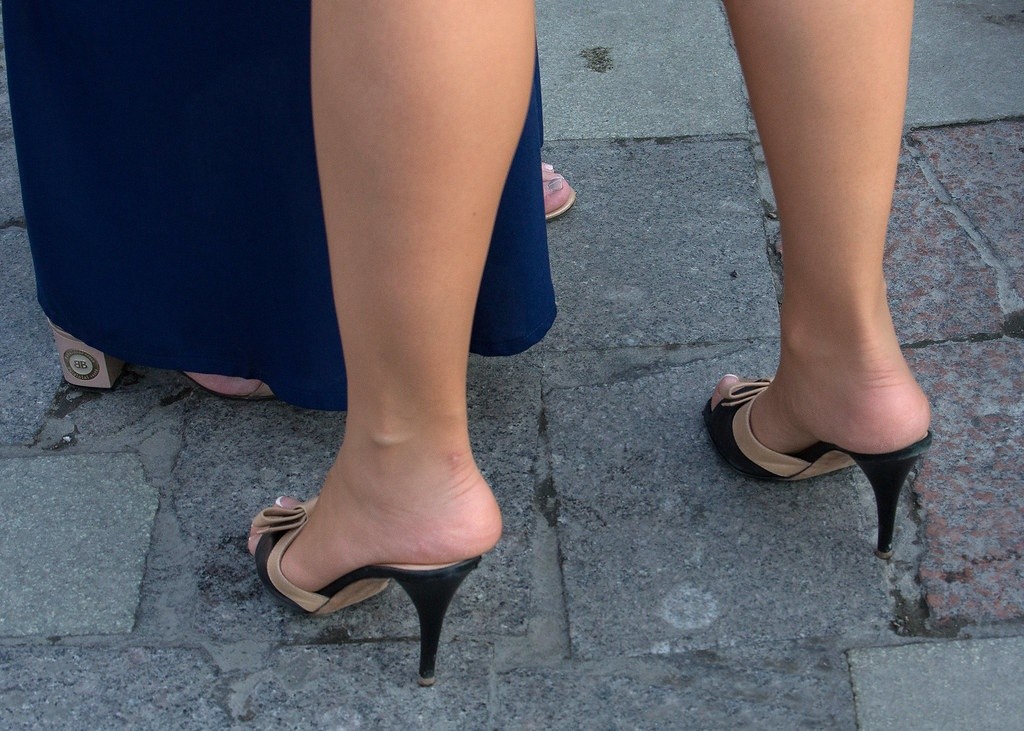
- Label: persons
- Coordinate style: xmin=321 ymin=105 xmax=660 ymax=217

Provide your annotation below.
xmin=3 ymin=0 xmax=578 ymax=408
xmin=246 ymin=0 xmax=930 ymax=685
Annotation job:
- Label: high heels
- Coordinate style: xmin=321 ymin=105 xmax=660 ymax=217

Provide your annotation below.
xmin=703 ymin=380 xmax=933 ymax=559
xmin=44 ymin=317 xmax=276 ymax=399
xmin=252 ymin=495 xmax=483 ymax=686
xmin=545 ymin=190 xmax=576 ymax=221
